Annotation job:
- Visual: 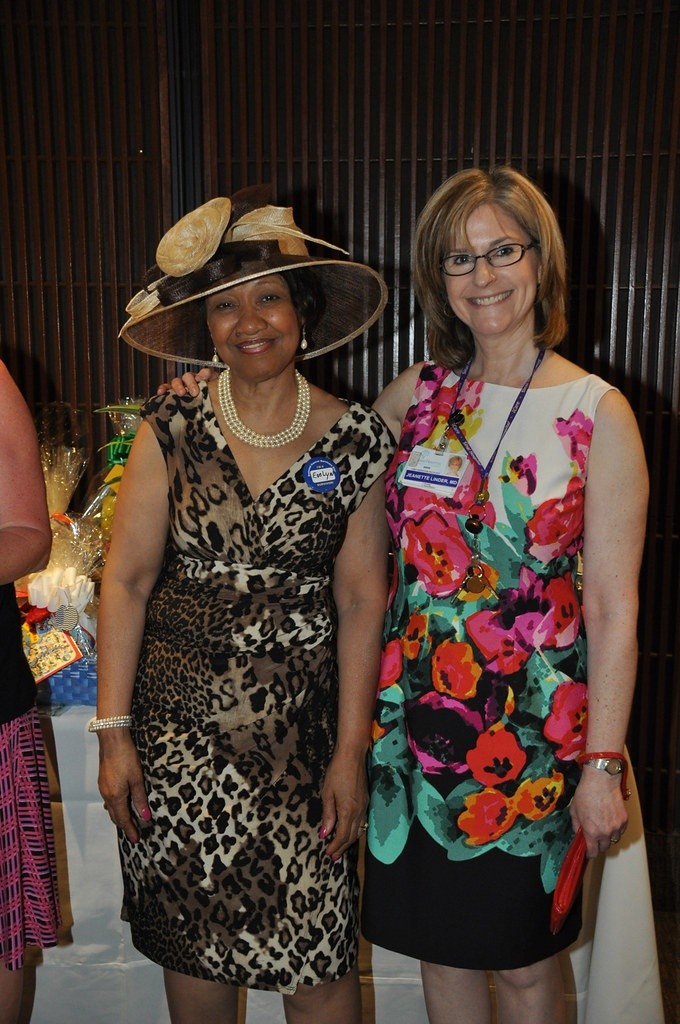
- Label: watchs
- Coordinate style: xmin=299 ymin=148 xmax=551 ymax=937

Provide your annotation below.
xmin=584 ymin=757 xmax=625 ymax=776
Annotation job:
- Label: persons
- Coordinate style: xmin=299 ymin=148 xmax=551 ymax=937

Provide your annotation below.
xmin=96 ymin=181 xmax=398 ymax=1024
xmin=448 ymin=456 xmax=462 ymax=476
xmin=158 ymin=167 xmax=651 ymax=1024
xmin=0 ymin=358 xmax=63 ymax=1024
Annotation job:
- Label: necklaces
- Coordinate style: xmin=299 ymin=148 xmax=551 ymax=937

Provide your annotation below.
xmin=218 ymin=367 xmax=312 ymax=448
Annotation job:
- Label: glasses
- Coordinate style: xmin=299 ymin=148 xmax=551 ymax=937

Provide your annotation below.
xmin=439 ymin=240 xmax=537 ymax=276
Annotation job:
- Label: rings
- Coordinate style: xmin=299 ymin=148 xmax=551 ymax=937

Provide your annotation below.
xmin=611 ymin=839 xmax=618 ymax=843
xmin=359 ymin=822 xmax=369 ymax=831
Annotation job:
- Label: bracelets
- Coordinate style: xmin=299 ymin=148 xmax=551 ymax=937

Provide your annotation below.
xmin=88 ymin=715 xmax=132 ymax=732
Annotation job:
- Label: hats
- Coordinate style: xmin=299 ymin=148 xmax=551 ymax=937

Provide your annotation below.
xmin=119 ymin=183 xmax=389 ymax=368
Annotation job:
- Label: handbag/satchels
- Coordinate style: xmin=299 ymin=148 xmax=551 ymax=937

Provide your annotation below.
xmin=550 ymin=751 xmax=631 ymax=935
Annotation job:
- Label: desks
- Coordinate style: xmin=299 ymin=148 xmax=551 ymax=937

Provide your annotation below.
xmin=31 ymin=701 xmax=665 ymax=1024
